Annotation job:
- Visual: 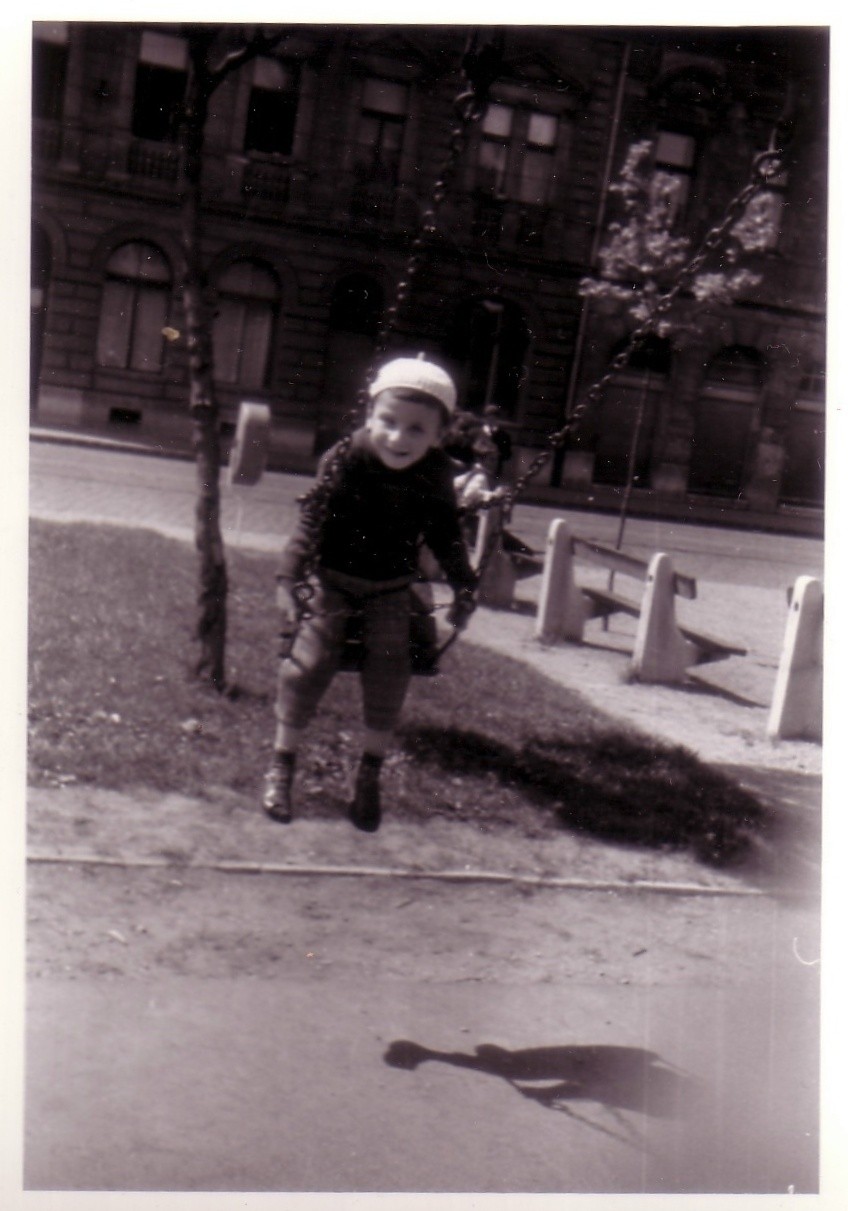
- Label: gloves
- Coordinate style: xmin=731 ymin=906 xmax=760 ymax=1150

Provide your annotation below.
xmin=276 ymin=577 xmax=309 ymax=624
xmin=446 ymin=590 xmax=477 ymax=627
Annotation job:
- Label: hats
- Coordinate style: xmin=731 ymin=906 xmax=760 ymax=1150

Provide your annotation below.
xmin=368 ymin=351 xmax=457 ymax=416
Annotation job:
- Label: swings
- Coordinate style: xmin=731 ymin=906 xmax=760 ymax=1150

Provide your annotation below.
xmin=277 ymin=67 xmax=798 ymax=675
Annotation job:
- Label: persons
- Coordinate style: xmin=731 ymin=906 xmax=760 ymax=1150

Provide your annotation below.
xmin=443 ymin=402 xmax=534 ymax=554
xmin=259 ymin=351 xmax=479 ymax=833
xmin=550 ymin=405 xmax=584 ymax=489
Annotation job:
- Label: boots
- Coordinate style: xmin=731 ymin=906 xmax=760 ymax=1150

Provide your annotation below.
xmin=349 ymin=752 xmax=384 ymax=832
xmin=264 ymin=747 xmax=299 ymax=822
